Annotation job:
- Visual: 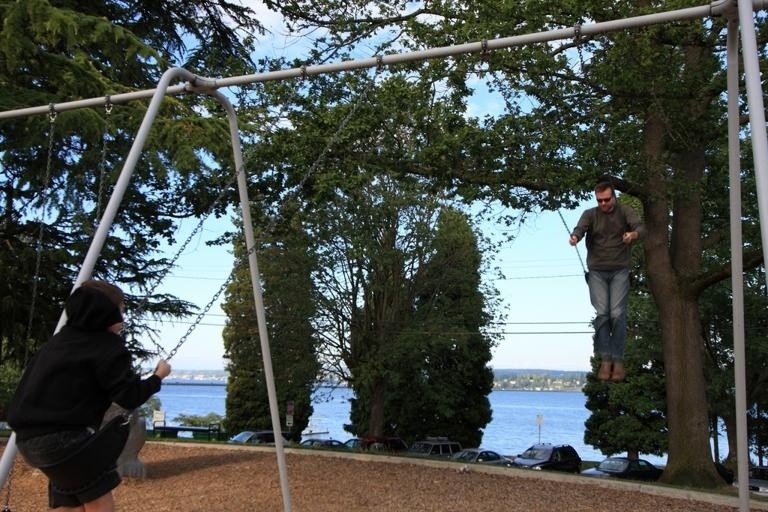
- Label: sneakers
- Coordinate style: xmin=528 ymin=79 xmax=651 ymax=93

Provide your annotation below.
xmin=596 ymin=361 xmax=626 ymax=382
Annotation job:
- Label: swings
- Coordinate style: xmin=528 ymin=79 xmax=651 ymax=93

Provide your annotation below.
xmin=0 ymin=93 xmax=113 ymax=512
xmin=481 ymin=22 xmax=636 ymax=289
xmin=37 ymin=55 xmax=382 ymax=490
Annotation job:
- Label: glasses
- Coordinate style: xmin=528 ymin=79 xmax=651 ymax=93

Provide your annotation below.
xmin=596 ymin=197 xmax=612 ymax=203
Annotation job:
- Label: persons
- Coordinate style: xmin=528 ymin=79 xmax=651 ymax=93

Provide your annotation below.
xmin=567 ymin=180 xmax=648 ymax=383
xmin=5 ymin=278 xmax=173 ymax=511
